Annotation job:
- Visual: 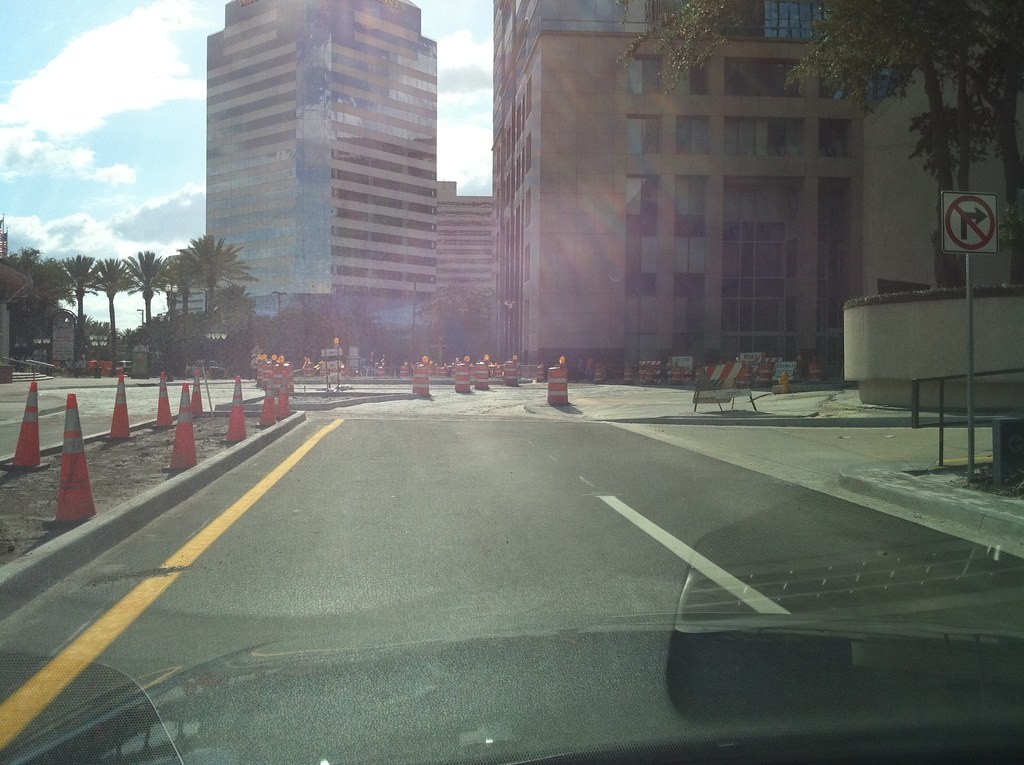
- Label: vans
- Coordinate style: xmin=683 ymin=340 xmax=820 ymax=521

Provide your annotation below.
xmin=116 ymin=361 xmax=131 ymax=376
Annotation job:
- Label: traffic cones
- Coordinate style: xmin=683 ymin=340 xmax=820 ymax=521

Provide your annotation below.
xmin=219 ymin=376 xmax=246 ymax=444
xmin=103 ymin=374 xmax=136 ymax=443
xmin=3 ymin=381 xmax=53 ymax=472
xmin=41 ymin=393 xmax=100 ymax=528
xmin=253 ymin=357 xmax=295 ymax=429
xmin=191 ymin=369 xmax=206 ymax=418
xmin=151 ymin=371 xmax=178 ymax=431
xmin=162 ymin=382 xmax=198 ymax=473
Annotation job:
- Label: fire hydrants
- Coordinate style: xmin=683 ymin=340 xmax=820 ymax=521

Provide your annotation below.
xmin=778 ymin=372 xmax=789 ymax=394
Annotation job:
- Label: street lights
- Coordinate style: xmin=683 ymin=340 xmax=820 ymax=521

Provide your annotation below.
xmin=271 ymin=291 xmax=287 ymax=310
xmin=136 ymin=309 xmax=144 ymax=325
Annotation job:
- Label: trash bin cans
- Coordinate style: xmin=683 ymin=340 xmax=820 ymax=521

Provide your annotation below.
xmin=94 ymin=367 xmax=101 ymax=378
xmin=992 ymin=418 xmax=1024 ymax=484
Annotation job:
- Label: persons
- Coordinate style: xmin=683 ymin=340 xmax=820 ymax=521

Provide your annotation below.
xmin=65 ymin=358 xmax=87 ymax=378
xmin=248 ymin=357 xmax=258 ymax=381
